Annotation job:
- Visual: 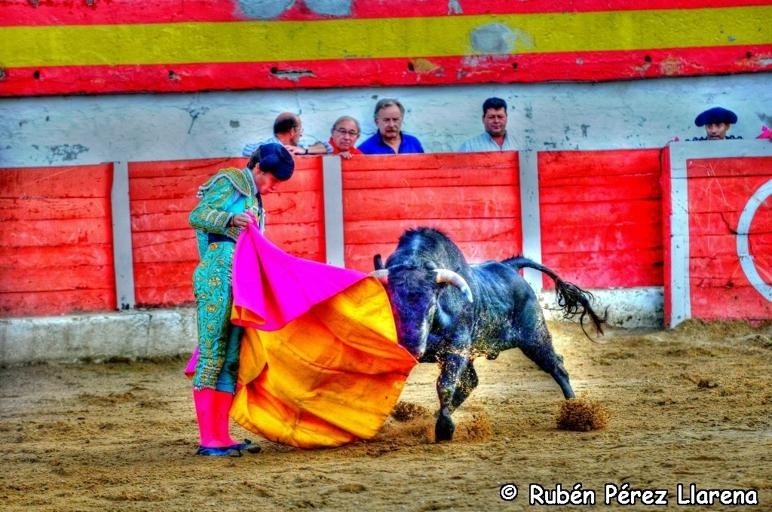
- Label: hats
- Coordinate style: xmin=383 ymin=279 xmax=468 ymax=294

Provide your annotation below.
xmin=256 ymin=142 xmax=294 ymax=180
xmin=695 ymin=106 xmax=737 ymax=127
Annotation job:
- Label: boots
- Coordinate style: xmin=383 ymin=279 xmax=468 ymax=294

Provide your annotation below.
xmin=192 ymin=386 xmax=261 ymax=456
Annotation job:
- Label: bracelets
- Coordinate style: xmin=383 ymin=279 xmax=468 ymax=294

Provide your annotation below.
xmin=232 ymin=216 xmax=238 ymax=227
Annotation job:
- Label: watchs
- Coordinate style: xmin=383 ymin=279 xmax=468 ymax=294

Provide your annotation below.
xmin=303 ymin=144 xmax=310 ymax=154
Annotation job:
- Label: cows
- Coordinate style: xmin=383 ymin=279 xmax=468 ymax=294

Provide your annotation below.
xmin=372 ymin=225 xmax=610 ymax=441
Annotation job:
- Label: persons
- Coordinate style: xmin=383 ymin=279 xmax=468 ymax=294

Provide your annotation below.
xmin=356 ymin=98 xmax=425 ymax=155
xmin=185 ymin=141 xmax=295 ymax=457
xmin=457 ymin=97 xmax=525 ymax=153
xmin=327 ymin=114 xmax=364 ymax=160
xmin=241 ymin=111 xmax=334 ymax=156
xmin=694 ymin=106 xmax=738 ymax=140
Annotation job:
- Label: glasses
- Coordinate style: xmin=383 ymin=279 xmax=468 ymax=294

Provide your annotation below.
xmin=335 ymin=127 xmax=357 ymax=137
